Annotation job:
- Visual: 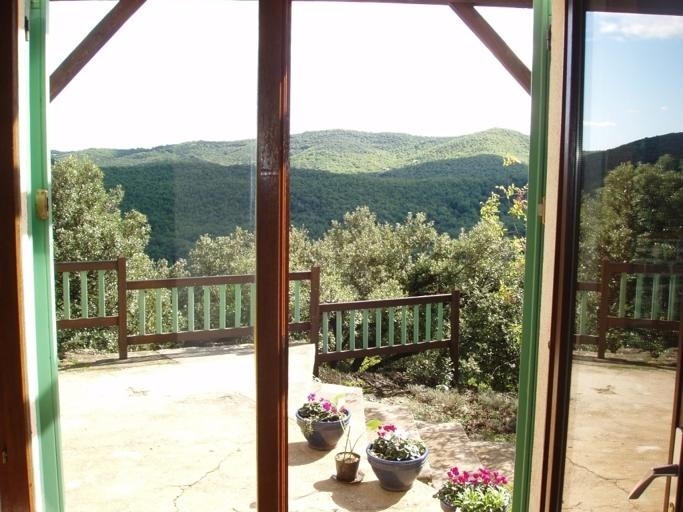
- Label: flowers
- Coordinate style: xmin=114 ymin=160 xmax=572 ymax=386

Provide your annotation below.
xmin=370 ymin=423 xmax=426 ymax=462
xmin=298 ymin=392 xmax=350 ymax=437
xmin=433 ymin=464 xmax=514 ymax=512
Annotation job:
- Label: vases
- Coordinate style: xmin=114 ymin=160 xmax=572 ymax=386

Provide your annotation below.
xmin=296 ymin=408 xmax=352 ymax=451
xmin=366 ymin=440 xmax=428 ymax=492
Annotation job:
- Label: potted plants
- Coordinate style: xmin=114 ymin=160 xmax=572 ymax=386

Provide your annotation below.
xmin=332 ymin=393 xmax=381 ymax=484
